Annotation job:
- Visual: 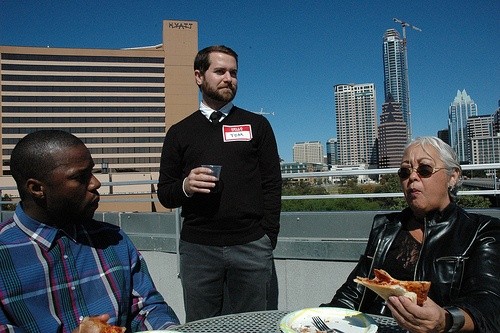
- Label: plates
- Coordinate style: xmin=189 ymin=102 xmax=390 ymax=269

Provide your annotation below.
xmin=279 ymin=307 xmax=378 ymax=333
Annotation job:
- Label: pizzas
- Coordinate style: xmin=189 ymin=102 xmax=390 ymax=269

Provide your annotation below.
xmin=353 ymin=269 xmax=431 ymax=306
xmin=78 ymin=316 xmax=126 ymax=333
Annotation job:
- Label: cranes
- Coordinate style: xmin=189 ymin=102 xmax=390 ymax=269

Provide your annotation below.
xmin=248 ymin=107 xmax=274 ymax=116
xmin=392 ymin=18 xmax=422 ymax=139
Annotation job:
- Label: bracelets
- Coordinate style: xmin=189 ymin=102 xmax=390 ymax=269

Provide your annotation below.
xmin=445 ymin=307 xmax=465 ymax=333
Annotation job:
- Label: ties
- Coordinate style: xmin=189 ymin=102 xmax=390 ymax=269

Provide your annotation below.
xmin=209 ymin=110 xmax=223 ymax=123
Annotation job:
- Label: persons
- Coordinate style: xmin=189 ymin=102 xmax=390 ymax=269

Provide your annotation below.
xmin=0 ymin=131 xmax=181 ymax=333
xmin=157 ymin=45 xmax=282 ymax=324
xmin=318 ymin=137 xmax=500 ymax=333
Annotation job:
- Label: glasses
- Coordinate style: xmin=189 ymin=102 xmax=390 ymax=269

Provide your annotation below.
xmin=398 ymin=165 xmax=450 ymax=180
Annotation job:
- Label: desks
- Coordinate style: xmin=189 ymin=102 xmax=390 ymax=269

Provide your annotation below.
xmin=168 ymin=309 xmax=407 ymax=333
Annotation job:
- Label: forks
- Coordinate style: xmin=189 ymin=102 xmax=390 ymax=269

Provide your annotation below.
xmin=312 ymin=316 xmax=345 ymax=333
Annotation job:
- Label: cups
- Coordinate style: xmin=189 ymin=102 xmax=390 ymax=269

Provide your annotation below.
xmin=202 ymin=165 xmax=222 ymax=191
